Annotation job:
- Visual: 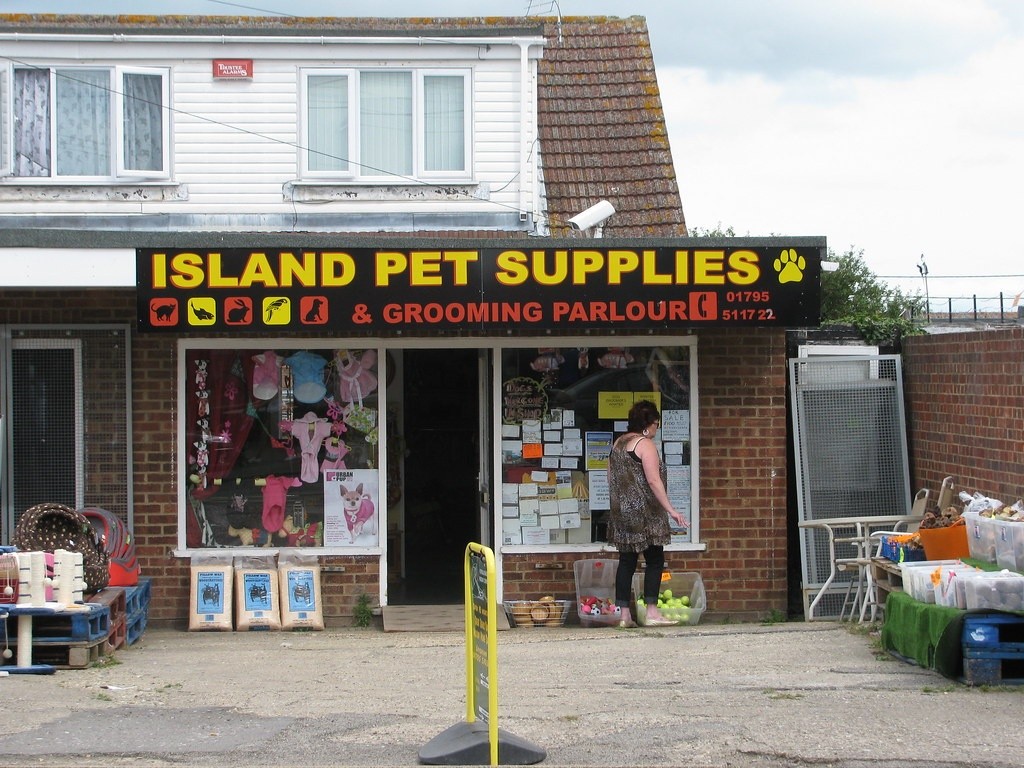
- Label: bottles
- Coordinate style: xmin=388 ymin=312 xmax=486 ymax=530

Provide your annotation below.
xmin=881 ymin=535 xmax=917 ymax=562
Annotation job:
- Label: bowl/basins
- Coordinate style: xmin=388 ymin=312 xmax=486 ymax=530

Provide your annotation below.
xmin=512 ymin=596 xmax=563 ymax=627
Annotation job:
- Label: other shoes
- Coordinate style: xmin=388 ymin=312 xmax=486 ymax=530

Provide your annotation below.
xmin=645 ymin=619 xmax=679 ymax=626
xmin=620 ymin=620 xmax=639 ymax=628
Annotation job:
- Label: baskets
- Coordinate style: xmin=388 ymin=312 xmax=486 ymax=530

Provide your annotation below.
xmin=881 ymin=537 xmax=924 ymax=563
xmin=503 ymin=600 xmax=572 ymax=628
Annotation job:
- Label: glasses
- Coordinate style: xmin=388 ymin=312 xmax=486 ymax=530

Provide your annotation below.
xmin=653 ymin=421 xmax=660 ymax=428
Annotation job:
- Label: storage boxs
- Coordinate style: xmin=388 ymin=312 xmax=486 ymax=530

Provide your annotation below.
xmin=972 ymin=577 xmax=1024 ymax=611
xmin=574 ymin=559 xmax=637 ymax=627
xmin=992 ymin=520 xmax=1024 ymax=572
xmin=909 ymin=563 xmax=973 ymax=601
xmin=953 ymin=571 xmax=1022 ymax=610
xmin=962 ymin=512 xmax=996 ymax=564
xmin=881 ymin=537 xmax=926 ymax=565
xmin=934 ymin=568 xmax=991 ymax=606
xmin=897 ymin=560 xmax=967 ymax=597
xmin=632 ymin=572 xmax=706 ymax=626
xmin=917 ymin=566 xmax=986 ymax=602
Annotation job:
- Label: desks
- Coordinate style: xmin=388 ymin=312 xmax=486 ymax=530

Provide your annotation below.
xmin=797 ymin=513 xmax=925 ymax=622
xmin=881 ymin=592 xmax=1024 ymax=687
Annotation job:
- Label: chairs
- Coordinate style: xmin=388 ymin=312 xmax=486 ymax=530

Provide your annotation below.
xmin=839 ymin=475 xmax=955 ymax=628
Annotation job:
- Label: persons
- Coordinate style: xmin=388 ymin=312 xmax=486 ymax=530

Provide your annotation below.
xmin=606 ymin=399 xmax=690 ymax=628
